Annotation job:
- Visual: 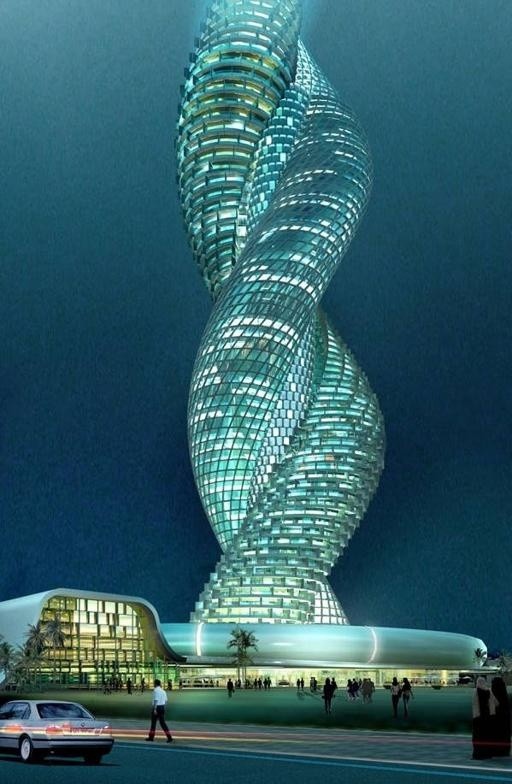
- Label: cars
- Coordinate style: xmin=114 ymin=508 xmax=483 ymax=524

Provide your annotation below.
xmin=1 ymin=700 xmax=115 ymax=765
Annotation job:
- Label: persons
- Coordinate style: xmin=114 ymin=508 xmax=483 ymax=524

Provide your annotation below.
xmin=324 ymin=678 xmax=337 ymax=713
xmin=145 ymin=679 xmax=175 ymax=743
xmin=391 ymin=677 xmax=413 ymax=717
xmin=314 ymin=680 xmax=317 ymax=690
xmin=470 ymin=675 xmax=512 ymax=762
xmin=168 ymin=680 xmax=172 ymax=689
xmin=102 ymin=677 xmax=145 ymax=695
xmin=181 ymin=680 xmax=214 ymax=687
xmin=297 ymin=678 xmax=304 ymax=689
xmin=347 ymin=678 xmax=375 ymax=704
xmin=227 ymin=679 xmax=233 ymax=697
xmin=235 ymin=677 xmax=271 ymax=689
